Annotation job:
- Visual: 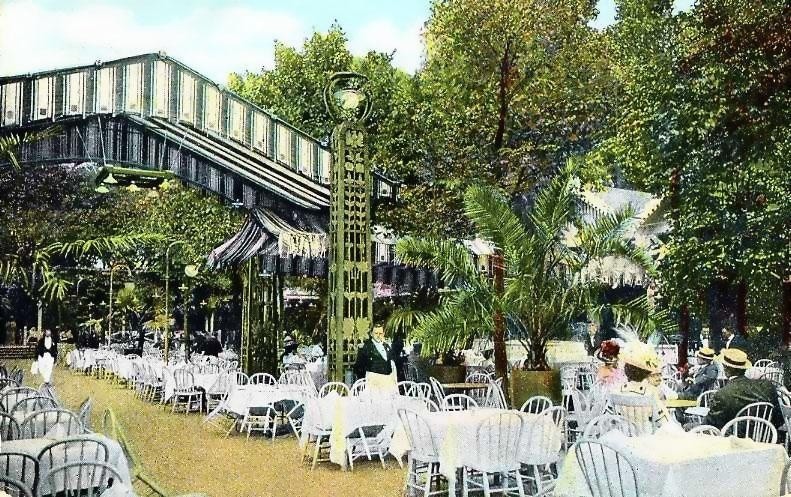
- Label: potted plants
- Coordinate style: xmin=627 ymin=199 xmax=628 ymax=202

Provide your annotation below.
xmin=382 ymin=157 xmax=684 ymax=413
xmin=388 ymin=296 xmax=476 ymax=403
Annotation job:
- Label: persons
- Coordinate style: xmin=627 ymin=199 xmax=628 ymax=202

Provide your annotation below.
xmin=353 ymin=322 xmax=393 ymax=381
xmin=645 ymin=351 xmax=677 ymax=400
xmin=701 ymin=346 xmax=785 ymax=444
xmin=33 ymin=327 xmax=59 ymax=386
xmin=612 ymin=339 xmax=672 ymax=430
xmin=716 ymin=324 xmax=749 ymax=353
xmin=584 ymin=321 xmax=602 ymax=356
xmin=595 ymin=337 xmax=621 ymax=406
xmin=671 ymin=346 xmax=718 ymax=428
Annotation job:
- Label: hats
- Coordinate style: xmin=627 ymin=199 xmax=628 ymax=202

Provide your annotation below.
xmin=697 ymin=347 xmax=714 ymax=361
xmin=716 ymin=349 xmax=752 ymax=371
xmin=594 ymin=336 xmax=624 ymax=364
xmin=617 ymin=343 xmax=662 ymax=373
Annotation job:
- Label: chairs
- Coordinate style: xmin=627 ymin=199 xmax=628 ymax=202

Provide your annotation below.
xmin=3 ymin=362 xmax=164 ymax=496
xmin=297 ymin=345 xmax=790 ymax=493
xmin=63 ymin=346 xmax=315 ymax=442
